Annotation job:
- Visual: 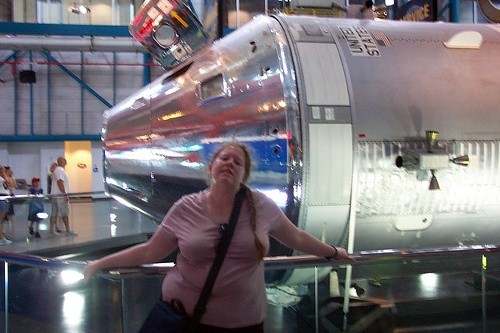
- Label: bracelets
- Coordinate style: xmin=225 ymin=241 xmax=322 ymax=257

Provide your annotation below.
xmin=325 ymin=246 xmax=337 ymax=259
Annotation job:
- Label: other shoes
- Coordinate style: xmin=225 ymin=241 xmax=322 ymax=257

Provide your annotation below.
xmin=28 ymin=227 xmax=34 ymax=235
xmin=35 ymin=232 xmax=41 ymax=238
xmin=47 ymin=232 xmax=60 ymax=238
xmin=0 ymin=238 xmax=12 ymax=246
xmin=65 ymin=231 xmax=78 ymax=236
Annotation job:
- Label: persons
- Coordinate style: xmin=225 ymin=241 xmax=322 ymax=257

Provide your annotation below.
xmin=50 ymin=157 xmax=78 ymax=236
xmin=81 ymin=142 xmax=357 ymax=332
xmin=0 ymin=165 xmax=17 ymax=244
xmin=28 ymin=176 xmax=45 ymax=238
xmin=47 ymin=163 xmax=62 ymax=232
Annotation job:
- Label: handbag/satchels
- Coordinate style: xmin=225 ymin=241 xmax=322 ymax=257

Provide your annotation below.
xmin=137 ymin=297 xmax=196 ymax=333
